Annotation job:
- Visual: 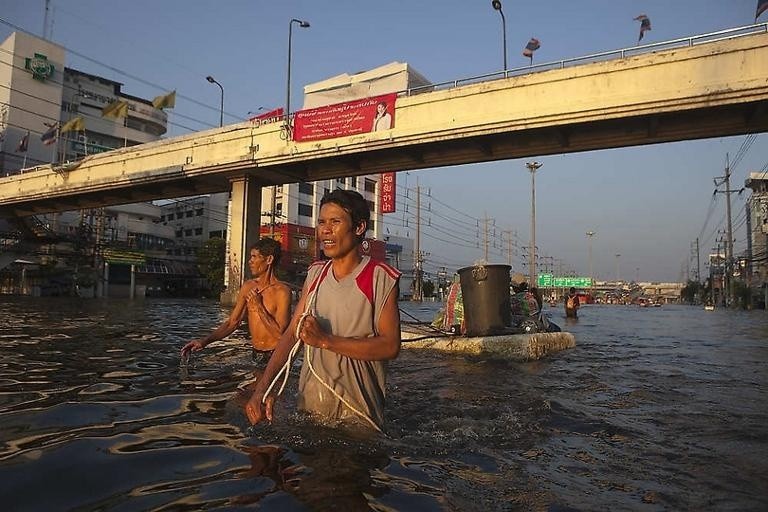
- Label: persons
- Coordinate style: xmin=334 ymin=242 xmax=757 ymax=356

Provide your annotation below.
xmin=508 ymin=273 xmax=561 ymax=333
xmin=564 ymin=287 xmax=580 ymax=317
xmin=370 ymin=102 xmax=391 ymax=131
xmin=178 ymin=236 xmax=292 ymax=363
xmin=243 ymin=187 xmax=403 ymax=431
xmin=244 ymin=416 xmax=391 ymax=511
xmin=531 ymin=288 xmax=543 ymax=310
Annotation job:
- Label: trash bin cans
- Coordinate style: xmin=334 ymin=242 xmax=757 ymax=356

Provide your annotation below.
xmin=457 ymin=265 xmax=512 ymax=337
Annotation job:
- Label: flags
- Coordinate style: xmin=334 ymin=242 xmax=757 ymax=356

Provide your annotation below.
xmin=519 ymin=37 xmax=542 ymax=59
xmin=151 ymin=88 xmax=176 ymax=110
xmin=632 ymin=13 xmax=653 ymax=43
xmin=13 ymin=132 xmax=29 ymax=154
xmin=59 ymin=114 xmax=85 ymax=133
xmin=98 ymin=99 xmax=128 ymax=120
xmin=755 ymin=1 xmax=767 ymax=20
xmin=39 ymin=120 xmax=57 ymax=146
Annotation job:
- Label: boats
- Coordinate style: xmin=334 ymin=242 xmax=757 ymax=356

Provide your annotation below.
xmin=401 ymin=318 xmax=580 ymax=362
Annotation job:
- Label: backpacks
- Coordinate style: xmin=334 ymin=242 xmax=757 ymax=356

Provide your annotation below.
xmin=566 ymin=295 xmax=577 ymax=309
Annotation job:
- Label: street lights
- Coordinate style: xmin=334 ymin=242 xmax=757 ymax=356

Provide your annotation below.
xmin=287 ymin=17 xmax=311 ymax=118
xmin=615 ymin=253 xmax=621 ymax=294
xmin=492 ymin=0 xmax=510 ymax=76
xmin=51 ymin=92 xmax=94 ymax=230
xmin=585 ymin=231 xmax=597 ymax=294
xmin=636 ymin=267 xmax=640 ymax=282
xmin=206 ymin=76 xmax=224 ymax=128
xmin=525 ymin=159 xmax=545 ymax=292
